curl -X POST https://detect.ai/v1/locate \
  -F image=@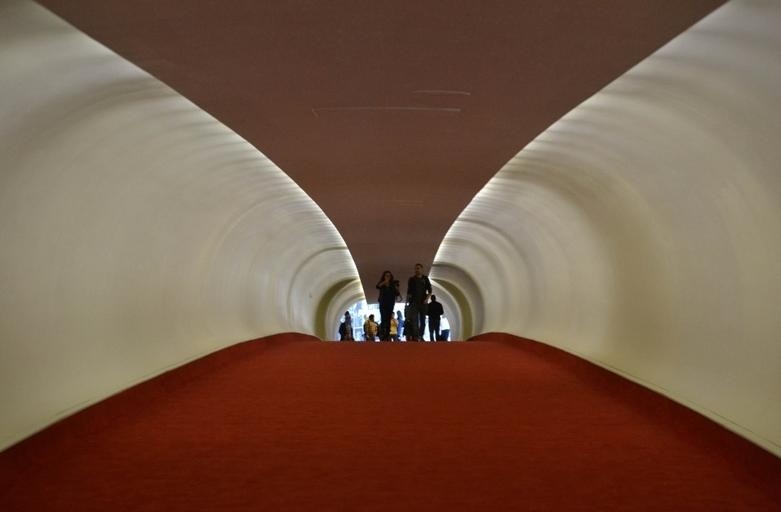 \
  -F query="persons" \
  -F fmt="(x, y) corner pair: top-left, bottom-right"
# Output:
(407, 263), (432, 342)
(339, 311), (405, 342)
(376, 271), (400, 342)
(428, 295), (443, 342)
